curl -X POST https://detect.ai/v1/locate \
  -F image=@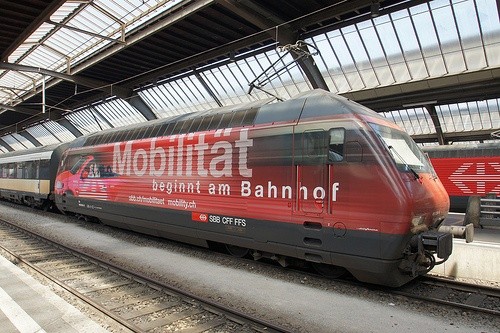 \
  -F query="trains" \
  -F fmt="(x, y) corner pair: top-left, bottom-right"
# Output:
(0, 89), (474, 288)
(419, 143), (499, 213)
